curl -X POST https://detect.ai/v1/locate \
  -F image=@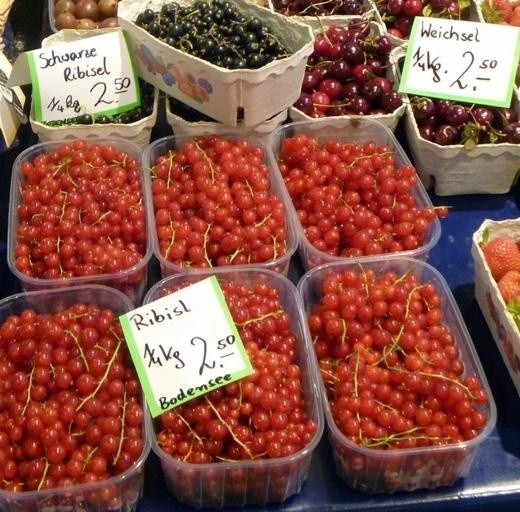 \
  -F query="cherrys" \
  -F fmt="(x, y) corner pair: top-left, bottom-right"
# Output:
(0, 0), (520, 511)
